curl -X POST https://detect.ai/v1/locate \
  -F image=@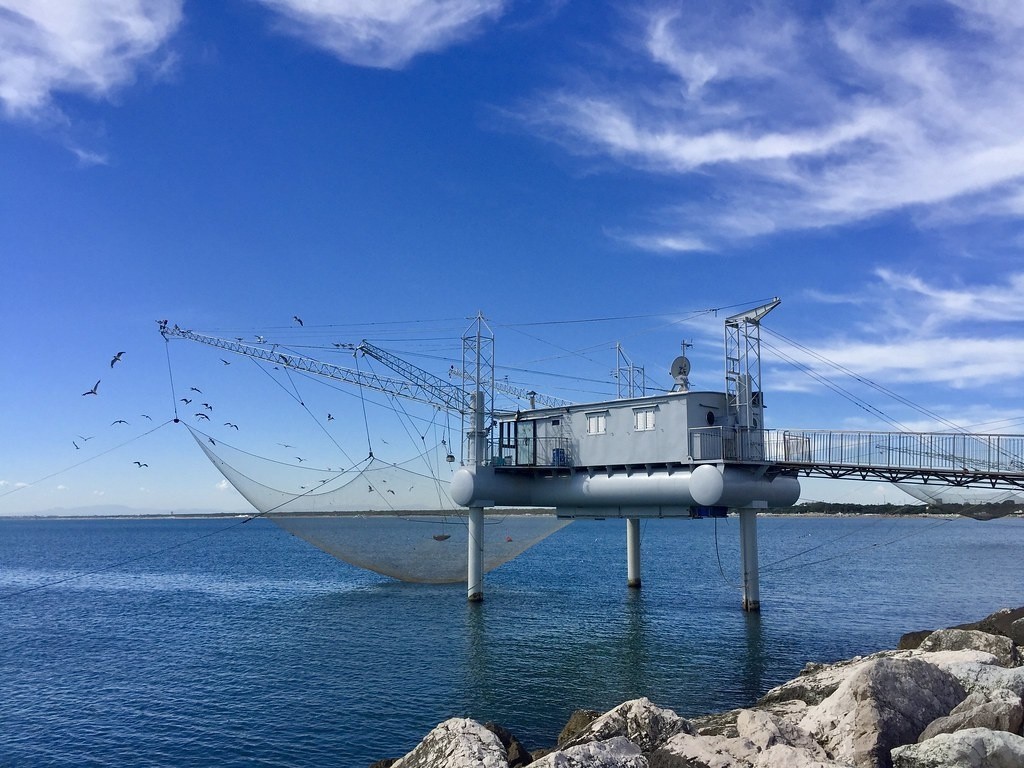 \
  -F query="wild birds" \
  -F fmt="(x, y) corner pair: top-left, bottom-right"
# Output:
(72, 315), (415, 494)
